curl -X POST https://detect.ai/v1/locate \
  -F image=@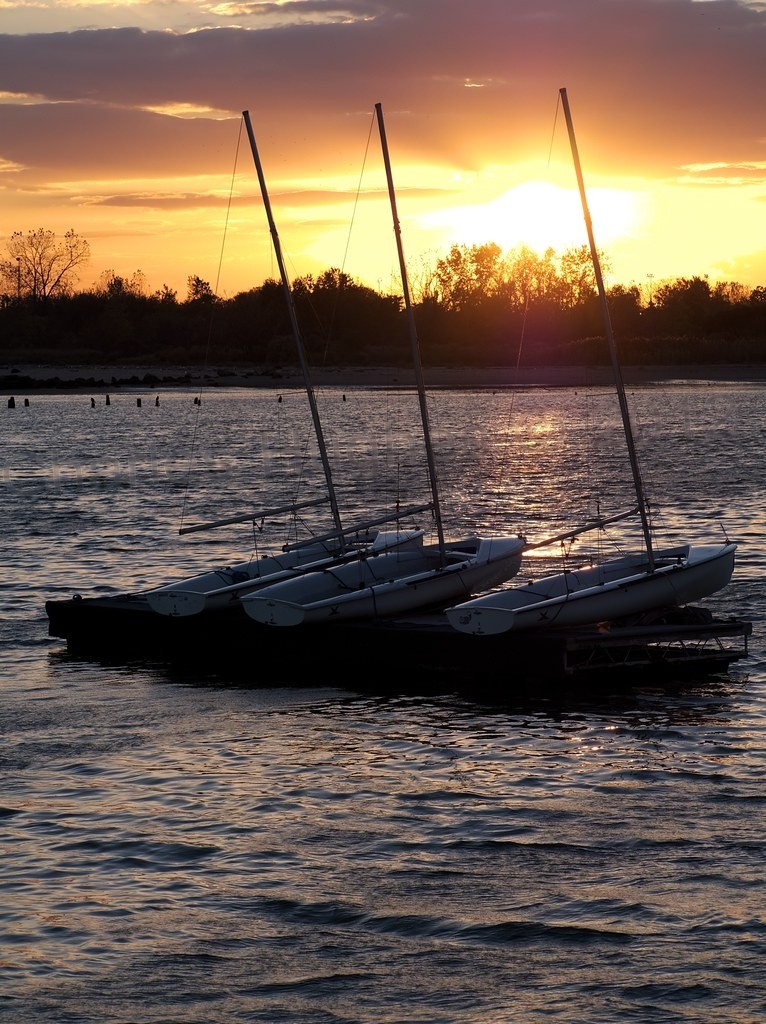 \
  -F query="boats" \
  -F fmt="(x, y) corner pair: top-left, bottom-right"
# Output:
(238, 103), (527, 627)
(130, 111), (426, 617)
(442, 87), (737, 636)
